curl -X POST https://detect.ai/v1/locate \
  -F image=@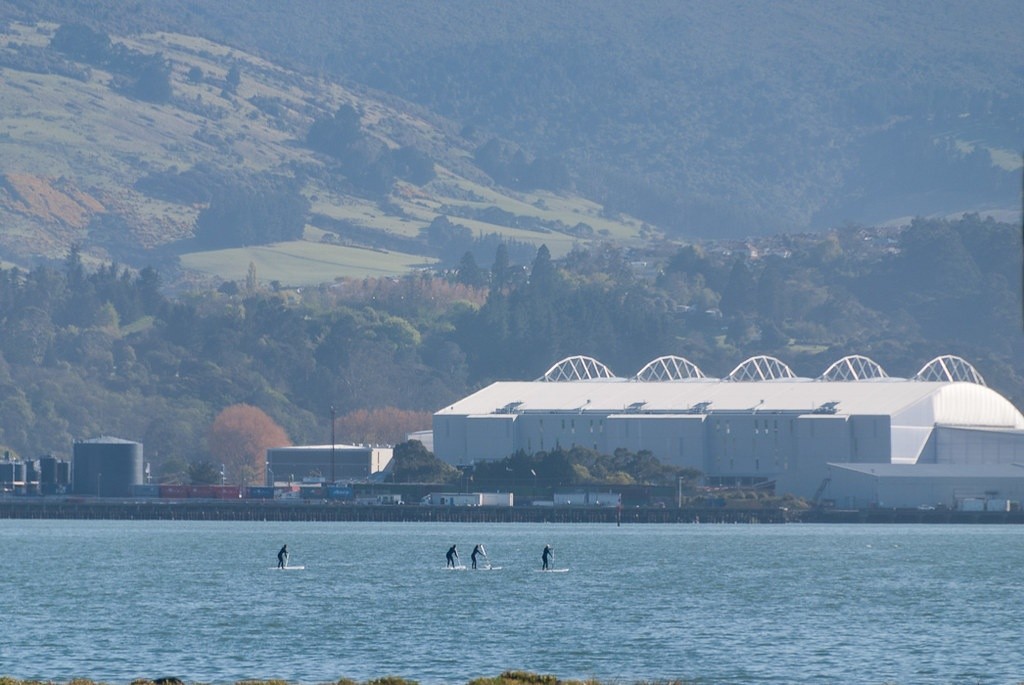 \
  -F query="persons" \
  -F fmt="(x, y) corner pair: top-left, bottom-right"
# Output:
(542, 544), (554, 570)
(278, 544), (288, 569)
(471, 544), (485, 570)
(446, 544), (458, 568)
